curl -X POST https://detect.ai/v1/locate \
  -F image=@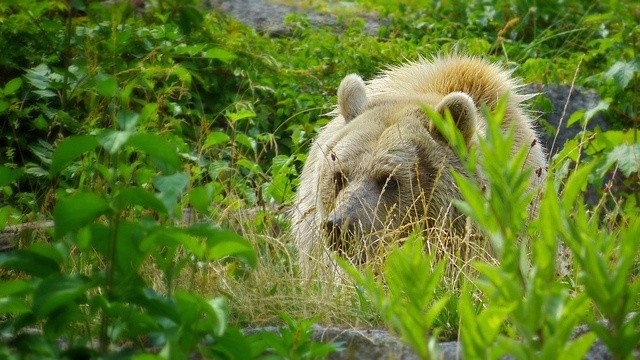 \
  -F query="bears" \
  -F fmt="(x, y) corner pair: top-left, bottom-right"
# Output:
(286, 51), (560, 324)
